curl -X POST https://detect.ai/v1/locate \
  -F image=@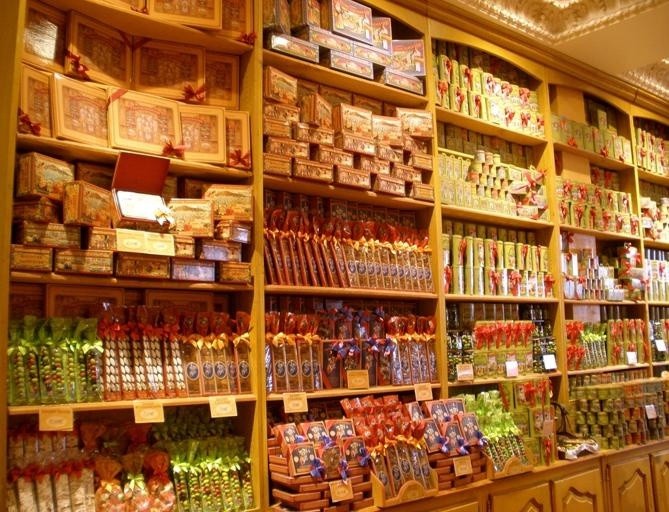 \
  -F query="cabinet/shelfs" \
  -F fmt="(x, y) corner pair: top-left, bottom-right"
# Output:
(631, 100), (669, 511)
(538, 63), (653, 512)
(428, 16), (570, 512)
(240, 0), (450, 510)
(0, 0), (265, 511)
